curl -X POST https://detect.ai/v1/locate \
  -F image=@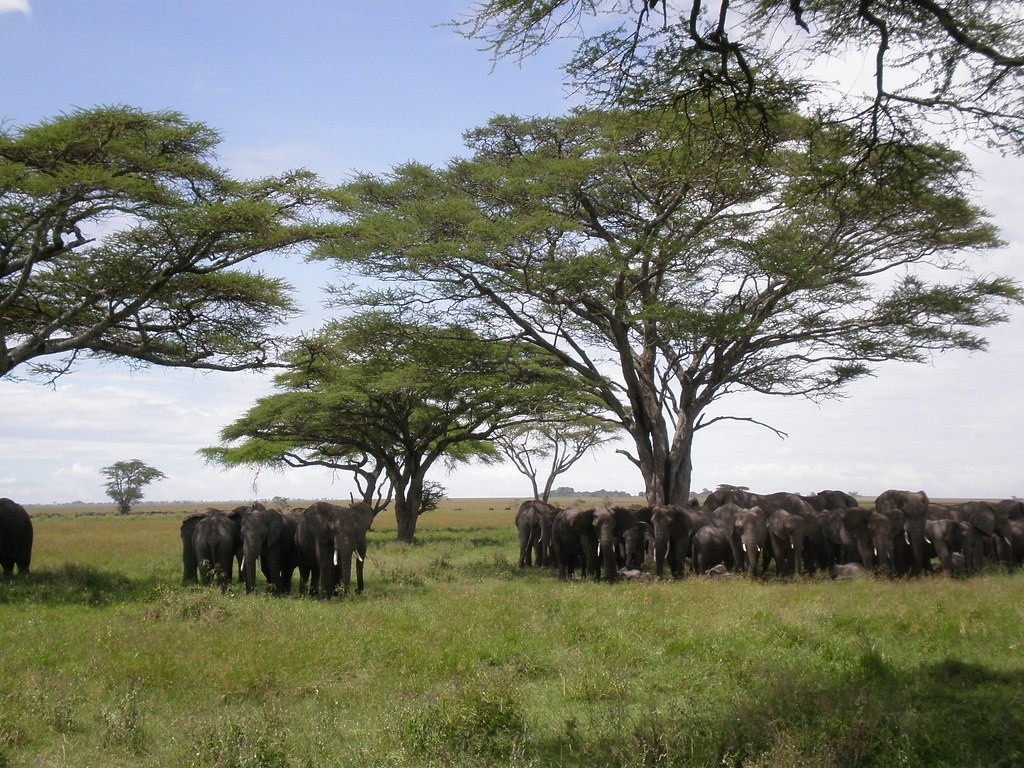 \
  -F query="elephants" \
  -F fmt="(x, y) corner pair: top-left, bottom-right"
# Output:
(514, 485), (1024, 586)
(0, 497), (34, 577)
(178, 500), (375, 600)
